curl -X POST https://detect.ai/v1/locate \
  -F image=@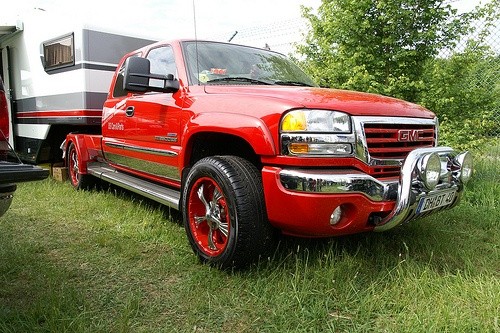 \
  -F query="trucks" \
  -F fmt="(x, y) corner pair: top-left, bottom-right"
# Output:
(1, 1), (472, 271)
